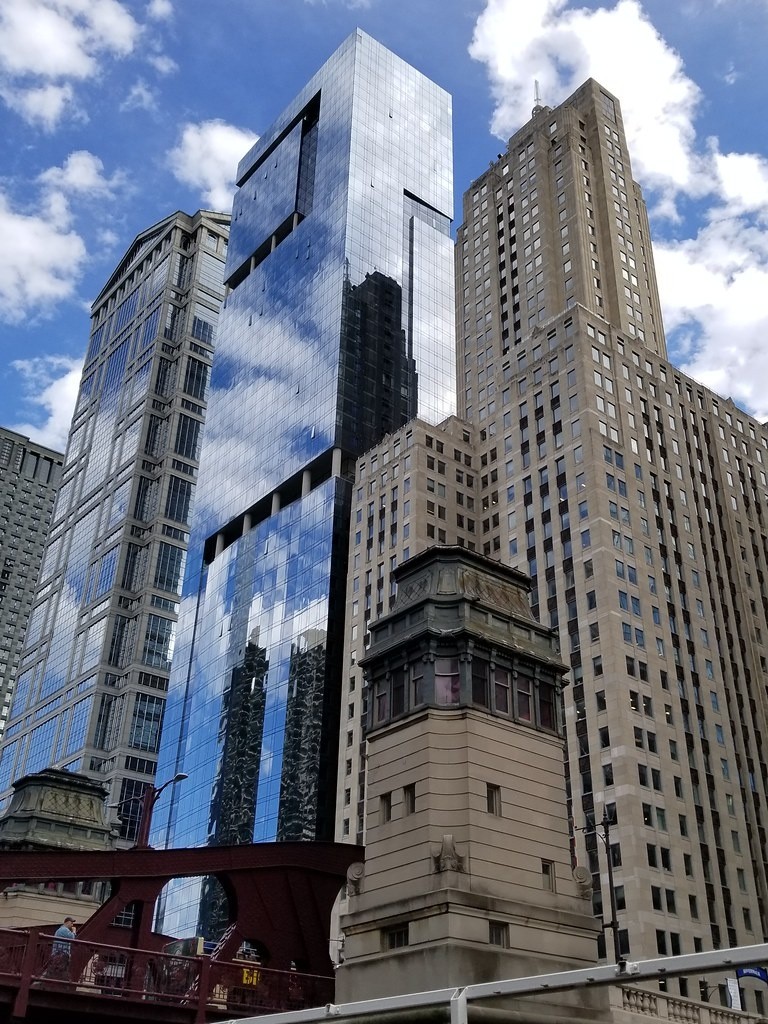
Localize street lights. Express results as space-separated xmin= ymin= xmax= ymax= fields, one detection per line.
xmin=106 ymin=771 xmax=189 ymax=851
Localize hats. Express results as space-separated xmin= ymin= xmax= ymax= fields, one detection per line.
xmin=64 ymin=916 xmax=77 ymax=923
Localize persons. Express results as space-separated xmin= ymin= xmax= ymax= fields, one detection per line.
xmin=32 ymin=917 xmax=77 ymax=985
